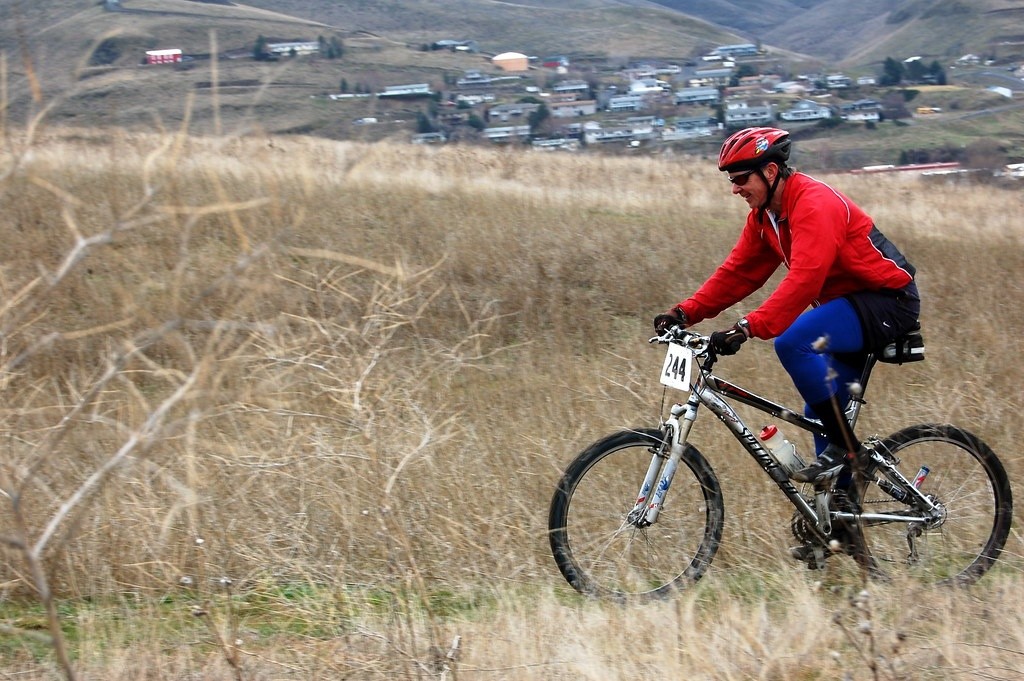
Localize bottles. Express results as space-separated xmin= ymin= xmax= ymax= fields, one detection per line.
xmin=760 ymin=425 xmax=807 ymax=477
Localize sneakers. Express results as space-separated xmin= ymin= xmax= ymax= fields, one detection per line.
xmin=791 ymin=443 xmax=868 ymax=483
xmin=790 ymin=543 xmax=836 ymax=562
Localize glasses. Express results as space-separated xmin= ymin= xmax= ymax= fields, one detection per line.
xmin=727 ymin=168 xmax=762 ymax=186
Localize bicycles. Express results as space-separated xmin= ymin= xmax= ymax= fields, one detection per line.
xmin=545 ymin=318 xmax=1013 ymax=606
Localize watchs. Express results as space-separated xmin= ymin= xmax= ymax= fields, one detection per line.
xmin=739 ymin=319 xmax=751 ymax=336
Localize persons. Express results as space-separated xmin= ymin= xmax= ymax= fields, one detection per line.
xmin=653 ymin=126 xmax=923 ymax=566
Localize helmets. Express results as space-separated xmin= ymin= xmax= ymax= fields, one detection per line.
xmin=719 ymin=127 xmax=789 ymax=172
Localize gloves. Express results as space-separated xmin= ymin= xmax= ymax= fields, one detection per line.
xmin=654 ymin=308 xmax=685 ymax=336
xmin=712 ymin=326 xmax=748 ymax=356
